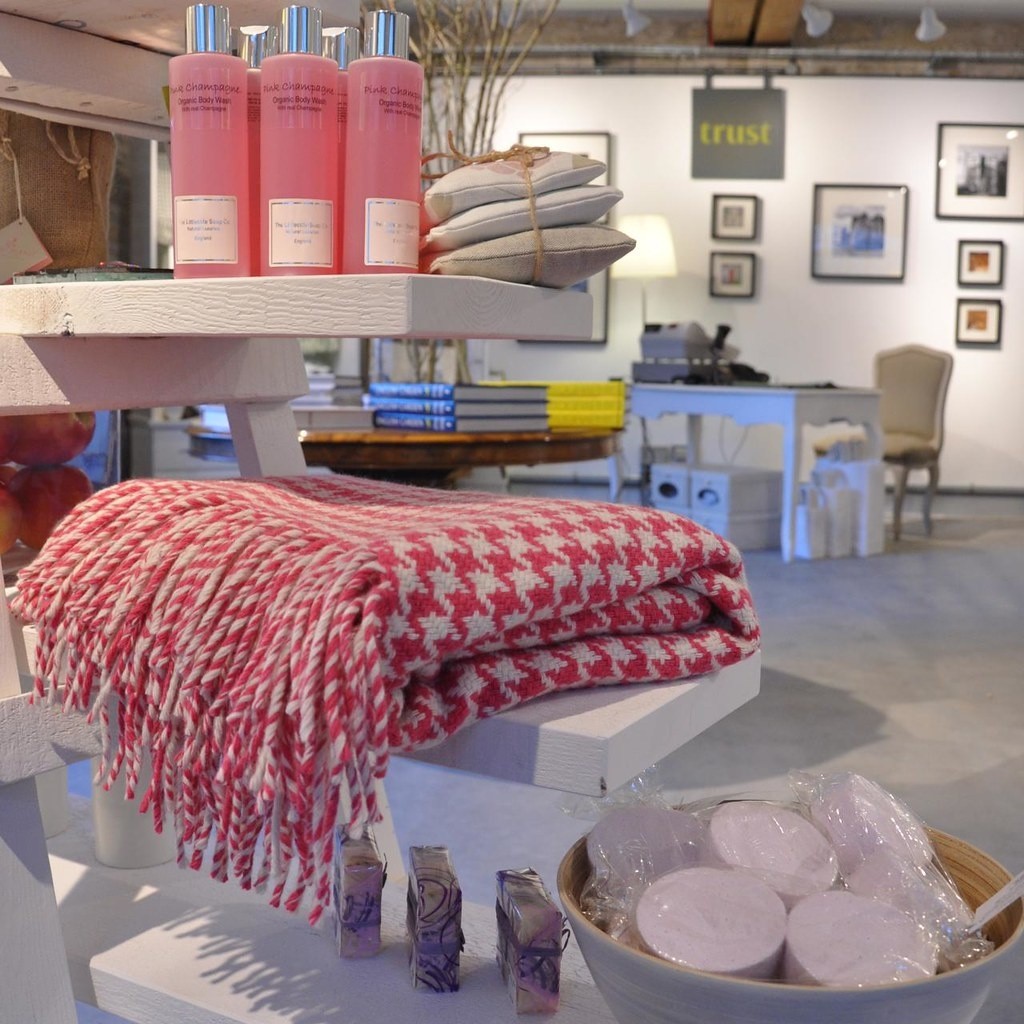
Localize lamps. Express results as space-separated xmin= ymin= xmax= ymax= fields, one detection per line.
xmin=609 ymin=214 xmax=676 ymax=445
xmin=915 ymin=5 xmax=945 ymax=42
xmin=620 ymin=0 xmax=650 ymax=37
xmin=801 ymin=4 xmax=832 ymax=35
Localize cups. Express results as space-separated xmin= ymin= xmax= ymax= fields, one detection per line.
xmin=637 ymin=868 xmax=786 ymax=982
xmin=700 ymin=802 xmax=839 ymax=914
xmin=785 ymin=890 xmax=936 ymax=987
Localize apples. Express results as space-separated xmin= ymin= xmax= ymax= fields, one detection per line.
xmin=0 ymin=412 xmax=96 ymax=557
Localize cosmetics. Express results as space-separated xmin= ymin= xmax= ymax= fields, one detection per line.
xmin=167 ymin=3 xmax=423 ymax=280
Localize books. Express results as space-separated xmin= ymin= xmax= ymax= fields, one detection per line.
xmin=192 ymin=374 xmax=625 ymax=435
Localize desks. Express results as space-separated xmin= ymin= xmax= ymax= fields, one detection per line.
xmin=607 ymin=384 xmax=884 ymax=561
xmin=185 ymin=421 xmax=615 ymax=491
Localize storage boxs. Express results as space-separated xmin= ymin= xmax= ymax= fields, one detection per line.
xmin=649 ymin=460 xmax=711 ymax=506
xmin=655 ymin=504 xmax=692 ymax=518
xmin=691 ymin=467 xmax=782 ymax=515
xmin=691 ymin=515 xmax=780 ymax=550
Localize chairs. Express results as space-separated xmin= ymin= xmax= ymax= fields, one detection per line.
xmin=812 ymin=344 xmax=953 ymax=542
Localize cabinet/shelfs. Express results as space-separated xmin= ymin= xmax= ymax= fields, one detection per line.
xmin=0 ymin=0 xmax=760 ymax=1023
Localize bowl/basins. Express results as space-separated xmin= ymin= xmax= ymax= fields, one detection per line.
xmin=556 ymin=803 xmax=1024 ymax=1024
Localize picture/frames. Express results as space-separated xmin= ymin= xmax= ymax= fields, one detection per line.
xmin=958 ymin=239 xmax=1004 ymax=286
xmin=955 ymin=297 xmax=1001 ymax=345
xmin=935 ymin=120 xmax=1024 ymax=223
xmin=810 ymin=183 xmax=907 ymax=280
xmin=709 ymin=252 xmax=756 ymax=299
xmin=517 ymin=130 xmax=611 ymax=344
xmin=710 ymin=193 xmax=757 ymax=239
